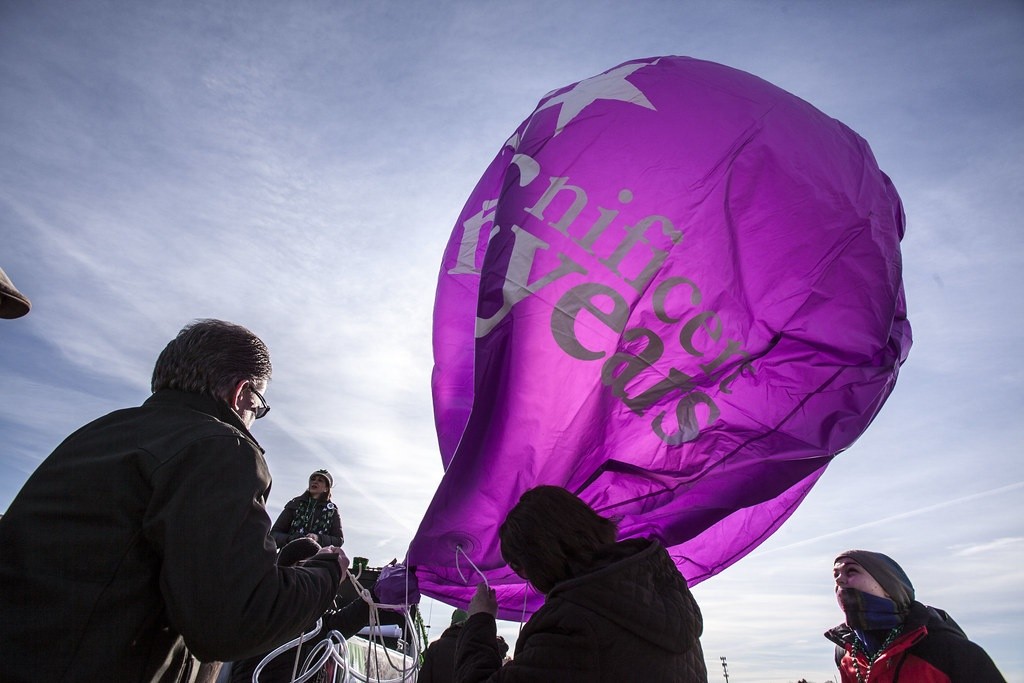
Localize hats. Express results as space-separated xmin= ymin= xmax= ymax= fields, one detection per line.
xmin=0 ymin=264 xmax=31 ymax=319
xmin=310 ymin=469 xmax=333 ymax=489
xmin=450 ymin=608 xmax=469 ymax=625
xmin=834 ymin=550 xmax=915 ymax=607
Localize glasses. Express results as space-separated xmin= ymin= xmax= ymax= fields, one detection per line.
xmin=236 ymin=379 xmax=270 ymax=419
xmin=509 ymin=561 xmax=530 ymax=580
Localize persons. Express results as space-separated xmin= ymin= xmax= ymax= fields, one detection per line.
xmin=236 ymin=538 xmax=398 ymax=682
xmin=417 ymin=607 xmax=473 ymax=682
xmin=2 ymin=317 xmax=351 ymax=683
xmin=444 ymin=485 xmax=709 ymax=683
xmin=822 ymin=550 xmax=1009 ymax=683
xmin=270 ymin=467 xmax=349 ymax=568
